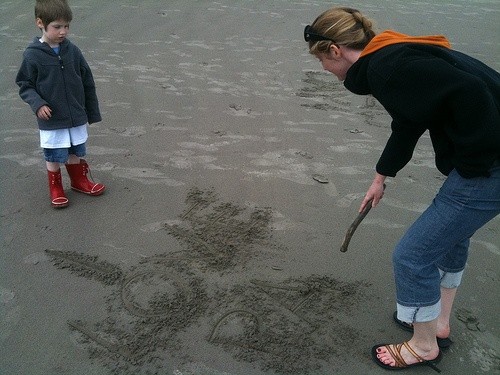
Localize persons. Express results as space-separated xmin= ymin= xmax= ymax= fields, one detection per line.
xmin=15 ymin=0 xmax=105 ymax=210
xmin=304 ymin=7 xmax=500 ymax=373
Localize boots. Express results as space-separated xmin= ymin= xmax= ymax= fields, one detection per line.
xmin=64 ymin=159 xmax=105 ymax=195
xmin=46 ymin=167 xmax=69 ymax=208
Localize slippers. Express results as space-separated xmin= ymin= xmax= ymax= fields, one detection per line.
xmin=371 ymin=341 xmax=442 ymax=370
xmin=393 ymin=311 xmax=451 ymax=348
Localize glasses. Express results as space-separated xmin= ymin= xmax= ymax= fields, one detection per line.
xmin=304 ymin=25 xmax=340 ymax=49
